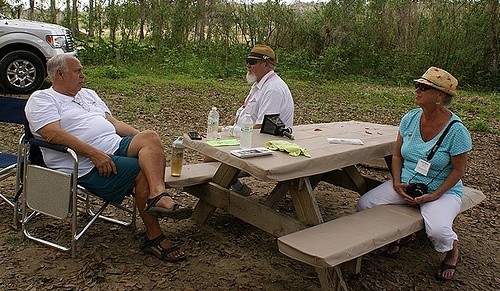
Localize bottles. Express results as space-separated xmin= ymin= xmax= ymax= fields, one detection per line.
xmin=171 ymin=136 xmax=185 ymax=177
xmin=221 ymin=126 xmax=241 ymax=137
xmin=206 ymin=107 xmax=219 ymax=141
xmin=240 ymin=113 xmax=253 ymax=150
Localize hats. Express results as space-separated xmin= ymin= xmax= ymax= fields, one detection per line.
xmin=414 ymin=66 xmax=458 ymax=97
xmin=243 ymin=44 xmax=276 ymax=66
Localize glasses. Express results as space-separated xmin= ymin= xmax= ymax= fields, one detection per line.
xmin=415 ymin=82 xmax=439 ymax=91
xmin=244 ymin=59 xmax=263 ymax=66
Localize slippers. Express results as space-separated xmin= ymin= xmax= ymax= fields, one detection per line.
xmin=435 ymin=251 xmax=461 ymax=280
xmin=383 ymin=233 xmax=412 ymax=257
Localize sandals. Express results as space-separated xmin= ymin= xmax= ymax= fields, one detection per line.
xmin=143 ymin=191 xmax=193 ymax=219
xmin=138 ymin=233 xmax=187 ymax=263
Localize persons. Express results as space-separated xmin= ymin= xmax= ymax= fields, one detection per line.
xmin=203 ymin=44 xmax=294 ymax=195
xmin=25 ymin=54 xmax=193 ymax=262
xmin=357 ymin=67 xmax=472 ymax=280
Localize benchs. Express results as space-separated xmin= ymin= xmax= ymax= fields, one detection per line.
xmin=277 ymin=184 xmax=486 ymax=291
xmin=161 ymin=162 xmax=255 ymax=227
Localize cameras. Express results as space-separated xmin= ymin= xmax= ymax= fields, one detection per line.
xmin=406 ymin=183 xmax=428 ymax=198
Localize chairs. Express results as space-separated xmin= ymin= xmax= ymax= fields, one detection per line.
xmin=0 ymin=97 xmax=137 ymax=258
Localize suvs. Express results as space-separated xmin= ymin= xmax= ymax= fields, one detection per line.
xmin=0 ymin=14 xmax=78 ymax=94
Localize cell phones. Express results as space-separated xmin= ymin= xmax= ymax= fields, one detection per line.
xmin=189 ymin=132 xmax=202 ymax=140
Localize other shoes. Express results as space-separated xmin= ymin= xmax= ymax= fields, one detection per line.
xmin=228 ymin=179 xmax=251 ymax=195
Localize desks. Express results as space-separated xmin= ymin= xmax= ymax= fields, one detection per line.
xmin=183 ymin=120 xmax=400 ymax=291
